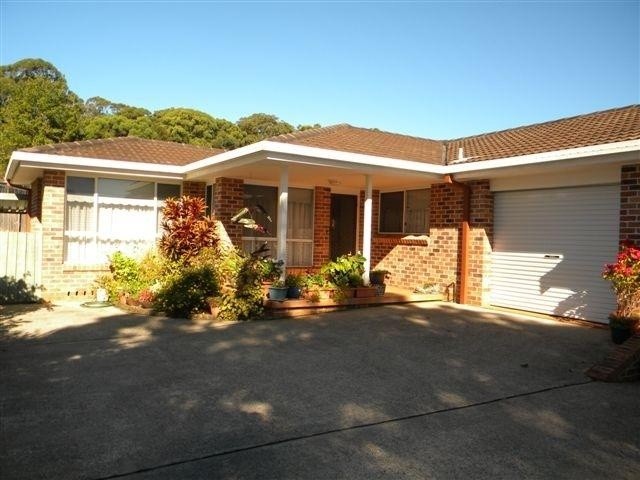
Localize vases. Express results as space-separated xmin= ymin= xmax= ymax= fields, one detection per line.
xmin=611 ymin=327 xmax=633 ymax=345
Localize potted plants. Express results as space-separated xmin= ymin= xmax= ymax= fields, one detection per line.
xmin=204 ymin=294 xmax=221 ymax=315
xmin=267 ymin=250 xmax=392 ymax=305
xmin=88 ymin=244 xmax=164 ymax=308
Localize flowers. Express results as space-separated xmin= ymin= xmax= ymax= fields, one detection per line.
xmin=600 ymin=238 xmax=640 ymax=334
